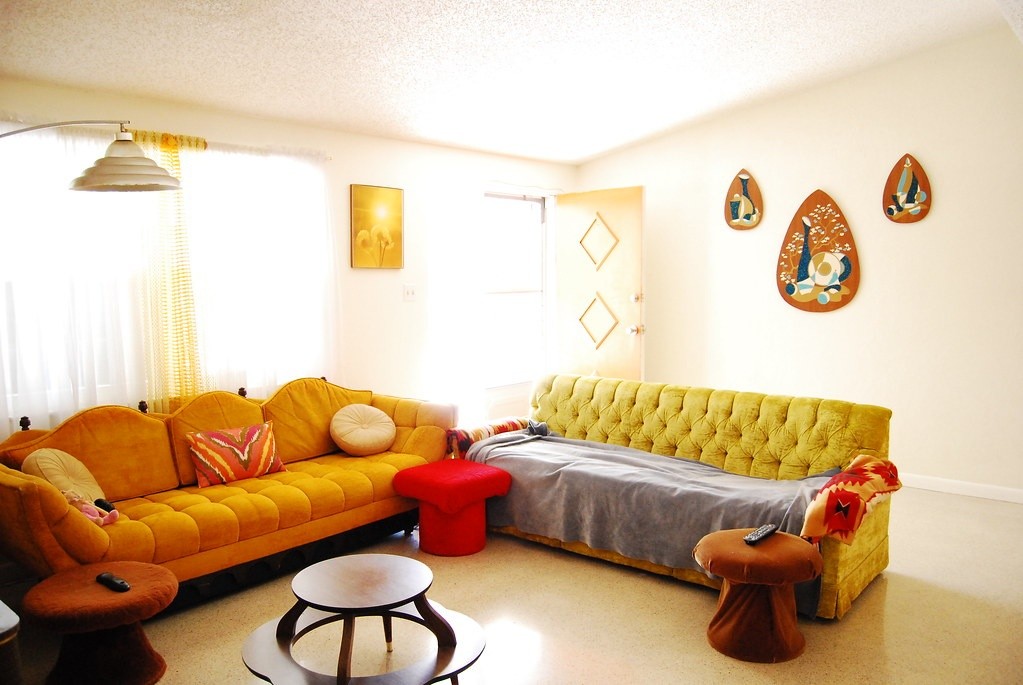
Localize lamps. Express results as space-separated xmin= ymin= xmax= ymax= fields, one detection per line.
xmin=0 ymin=119 xmax=183 ymax=191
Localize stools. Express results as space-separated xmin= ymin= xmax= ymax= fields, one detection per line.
xmin=391 ymin=460 xmax=511 ymax=557
xmin=690 ymin=528 xmax=826 ymax=662
xmin=22 ymin=561 xmax=179 ymax=685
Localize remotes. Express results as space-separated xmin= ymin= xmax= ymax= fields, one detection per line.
xmin=743 ymin=524 xmax=777 ymax=545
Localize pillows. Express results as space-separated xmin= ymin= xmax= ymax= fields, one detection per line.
xmin=330 ymin=403 xmax=398 ymax=457
xmin=25 ymin=446 xmax=106 ymax=514
xmin=183 ymin=419 xmax=285 ymax=487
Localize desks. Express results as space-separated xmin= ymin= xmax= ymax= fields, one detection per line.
xmin=243 ymin=552 xmax=487 ymax=685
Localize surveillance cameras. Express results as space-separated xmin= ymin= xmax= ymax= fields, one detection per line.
xmin=96 ymin=571 xmax=131 ymax=592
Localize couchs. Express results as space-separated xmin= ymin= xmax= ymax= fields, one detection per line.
xmin=444 ymin=372 xmax=891 ymax=617
xmin=0 ymin=378 xmax=456 ymax=582
